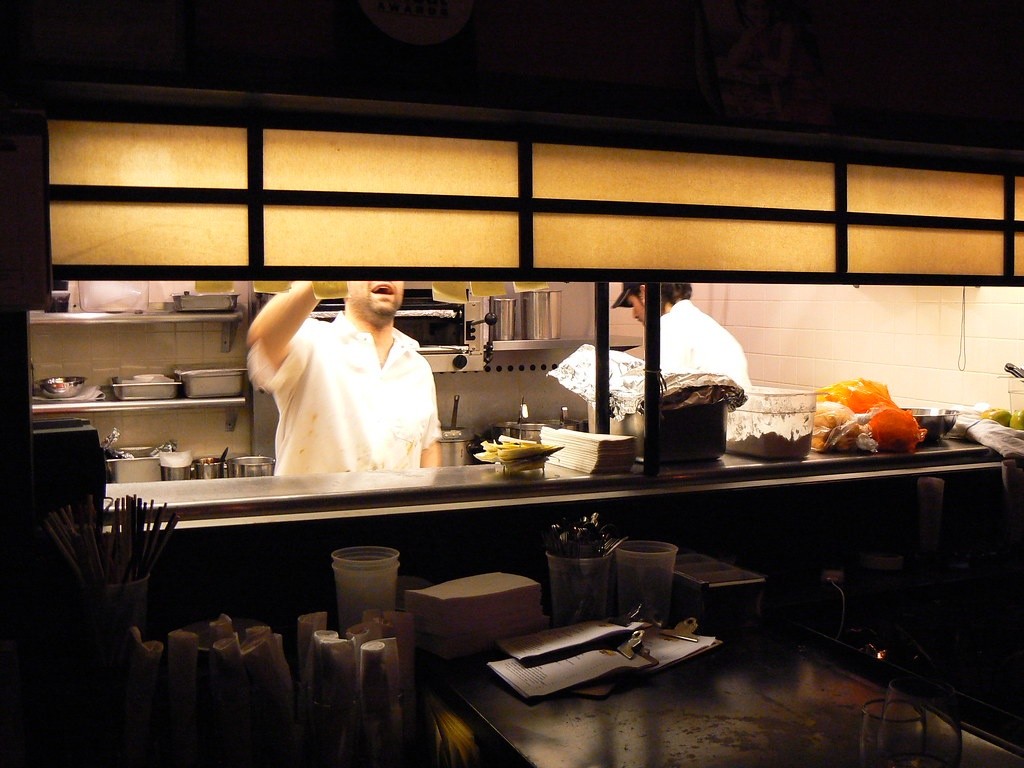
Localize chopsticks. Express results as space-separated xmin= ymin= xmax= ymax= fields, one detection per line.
xmin=43 ymin=494 xmax=181 ymax=586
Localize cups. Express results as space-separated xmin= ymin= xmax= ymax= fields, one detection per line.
xmin=81 ymin=573 xmax=149 ymax=663
xmin=859 ymin=678 xmax=963 ymax=768
xmin=546 ymin=548 xmax=613 ymax=628
xmin=331 ymin=546 xmax=401 ymax=638
xmin=616 ymin=540 xmax=679 ymax=627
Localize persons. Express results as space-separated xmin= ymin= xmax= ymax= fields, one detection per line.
xmin=610 ymin=283 xmax=754 ymax=393
xmin=244 ymin=280 xmax=444 ymax=477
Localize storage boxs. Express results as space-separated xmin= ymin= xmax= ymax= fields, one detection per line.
xmin=725 ymin=384 xmax=830 ymax=463
xmin=671 ymin=552 xmax=767 ymax=639
xmin=587 ymin=395 xmax=727 ymax=468
xmin=174 ymin=368 xmax=245 ymax=399
xmin=112 ymin=381 xmax=182 ymax=400
xmin=172 ymin=294 xmax=240 ymax=311
xmin=106 ymin=458 xmax=162 ymax=482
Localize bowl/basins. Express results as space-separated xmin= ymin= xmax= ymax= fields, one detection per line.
xmin=903 ymin=407 xmax=962 ymax=443
xmin=33 ymin=376 xmax=88 ymax=399
xmin=119 ymin=447 xmax=154 ymax=458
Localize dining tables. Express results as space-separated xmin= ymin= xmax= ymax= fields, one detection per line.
xmin=404 ymin=612 xmax=1024 ymax=767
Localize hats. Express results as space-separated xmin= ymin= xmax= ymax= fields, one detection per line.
xmin=612 ymin=280 xmax=644 ymax=311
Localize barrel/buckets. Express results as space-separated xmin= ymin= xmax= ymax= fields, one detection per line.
xmin=161 ymin=462 xmax=193 ymax=481
xmin=192 ymin=458 xmax=227 ymax=479
xmin=488 ymin=418 xmax=589 ymax=441
xmin=440 ymin=426 xmax=480 ymax=467
xmin=493 ymin=298 xmax=516 ymax=341
xmin=227 ymin=456 xmax=275 ymax=478
xmin=519 ymin=291 xmax=561 ymax=340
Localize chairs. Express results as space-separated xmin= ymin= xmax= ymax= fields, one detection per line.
xmin=817 ymin=475 xmax=945 ymax=676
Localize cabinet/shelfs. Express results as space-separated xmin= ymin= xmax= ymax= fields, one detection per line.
xmin=28 ymin=281 xmax=257 ymax=482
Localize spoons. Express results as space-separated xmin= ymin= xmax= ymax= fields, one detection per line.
xmin=543 ymin=511 xmax=628 ymax=560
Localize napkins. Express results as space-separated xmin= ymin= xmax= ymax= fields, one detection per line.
xmin=539 ymin=426 xmax=637 ymax=475
